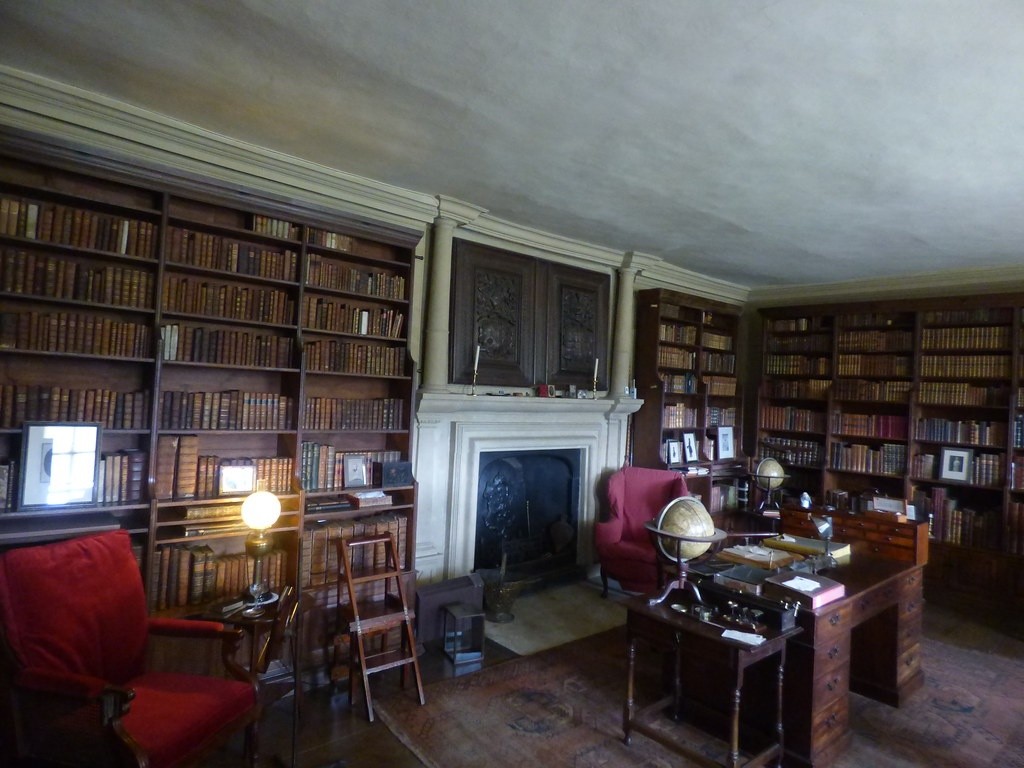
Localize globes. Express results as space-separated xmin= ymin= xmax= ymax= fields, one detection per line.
xmin=643 ymin=496 xmax=728 ymax=607
xmin=747 ymin=458 xmax=791 ymax=511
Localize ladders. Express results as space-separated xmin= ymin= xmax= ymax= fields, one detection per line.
xmin=335 ymin=530 xmax=425 ymax=723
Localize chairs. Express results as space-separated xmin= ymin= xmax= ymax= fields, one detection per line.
xmin=596 ymin=469 xmax=722 ymax=597
xmin=0 ymin=528 xmax=265 ymax=767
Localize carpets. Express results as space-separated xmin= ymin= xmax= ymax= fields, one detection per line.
xmin=369 ymin=622 xmax=1024 ymax=767
xmin=485 ymin=579 xmax=627 ymax=657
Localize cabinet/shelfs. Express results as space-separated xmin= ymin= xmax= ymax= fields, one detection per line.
xmin=636 ymin=289 xmax=747 ymax=512
xmin=1 ymin=146 xmax=414 ymax=695
xmin=780 ymin=503 xmax=930 ymax=567
xmin=752 ymin=290 xmax=1024 ymax=581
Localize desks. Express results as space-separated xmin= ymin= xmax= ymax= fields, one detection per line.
xmin=623 ymin=605 xmax=804 ymax=768
xmin=214 ymin=591 xmax=313 ymax=768
xmin=700 ymin=552 xmax=930 ymax=768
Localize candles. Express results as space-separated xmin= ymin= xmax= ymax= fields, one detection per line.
xmin=472 ymin=345 xmax=481 ymax=372
xmin=593 ymin=358 xmax=599 ymax=381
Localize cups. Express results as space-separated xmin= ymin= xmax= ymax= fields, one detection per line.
xmin=700 ymin=607 xmax=712 ymax=623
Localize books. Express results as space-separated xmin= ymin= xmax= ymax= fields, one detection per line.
xmin=0 ymin=195 xmax=405 ymax=612
xmin=655 ymin=307 xmax=1024 ymax=544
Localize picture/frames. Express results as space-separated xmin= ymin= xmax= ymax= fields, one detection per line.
xmin=716 ymin=424 xmax=735 ymax=461
xmin=15 ymin=421 xmax=102 ymax=512
xmin=681 ymin=431 xmax=699 ymax=463
xmin=667 ymin=440 xmax=681 ymax=466
xmin=342 ymin=454 xmax=369 ymax=488
xmin=218 ymin=465 xmax=257 ymax=497
xmin=937 ymin=446 xmax=975 ymax=486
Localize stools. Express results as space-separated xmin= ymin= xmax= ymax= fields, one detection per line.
xmin=441 ymin=602 xmax=489 ymax=665
